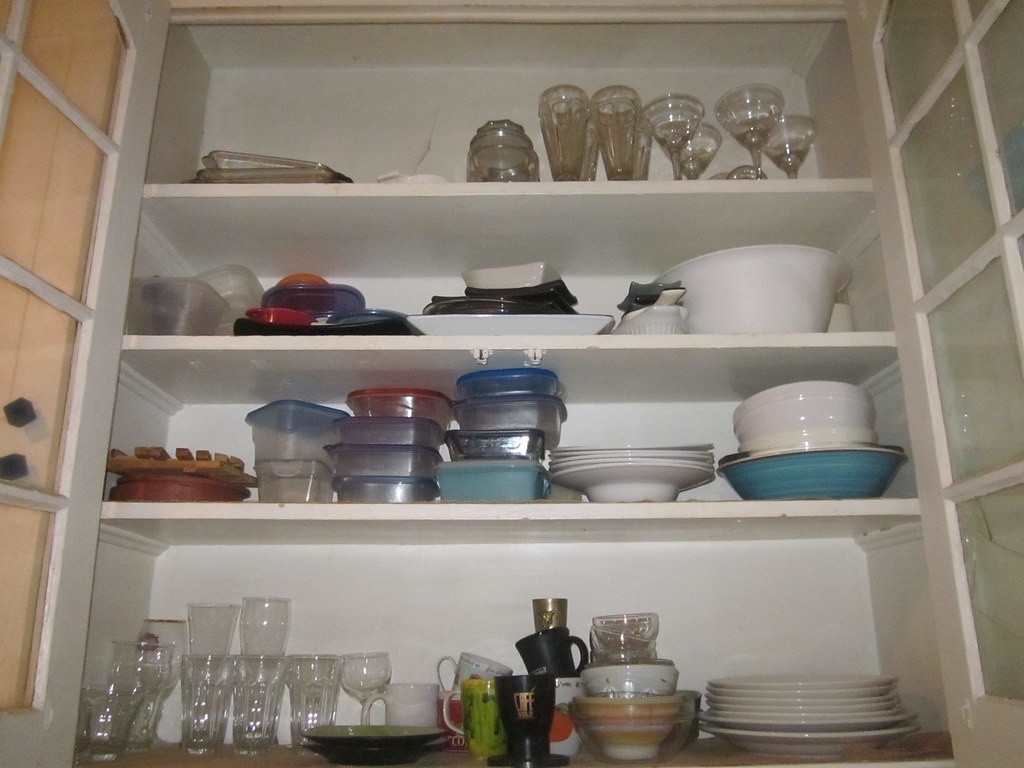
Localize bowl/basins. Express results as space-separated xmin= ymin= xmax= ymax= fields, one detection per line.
xmin=717 ymin=441 xmax=909 ymax=500
xmin=122 ymin=264 xmax=414 ymax=335
xmin=567 ymin=611 xmax=702 ymax=767
xmin=547 ymin=441 xmax=717 ymax=504
xmin=732 ymin=380 xmax=877 ymax=454
xmin=651 ymin=243 xmax=853 ymax=335
xmin=461 ymin=261 xmax=561 ymax=289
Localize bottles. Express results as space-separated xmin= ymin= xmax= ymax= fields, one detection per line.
xmin=466 ymin=119 xmax=541 ymax=182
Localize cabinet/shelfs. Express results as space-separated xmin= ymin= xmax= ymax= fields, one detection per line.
xmin=75 ymin=9 xmax=953 ymax=766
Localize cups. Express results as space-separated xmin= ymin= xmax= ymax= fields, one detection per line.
xmin=359 ymin=597 xmax=589 ymax=768
xmin=73 ymin=595 xmax=346 ymax=768
xmin=538 ymin=84 xmax=654 ymax=182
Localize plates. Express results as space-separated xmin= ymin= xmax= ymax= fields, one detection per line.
xmin=404 ymin=279 xmax=616 ymax=336
xmin=189 ymin=148 xmax=354 ymax=184
xmin=606 ymin=280 xmax=689 ymax=335
xmin=298 ymin=724 xmax=454 ymax=766
xmin=697 ymin=670 xmax=921 ymax=760
xmin=232 ymin=316 xmax=412 ymax=336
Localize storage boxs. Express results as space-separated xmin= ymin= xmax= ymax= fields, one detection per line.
xmin=126 ymin=268 xmax=227 ymax=337
xmin=322 ymin=388 xmax=453 ymax=503
xmin=190 ymin=264 xmax=267 ymax=316
xmin=436 ymin=368 xmax=569 ymax=503
xmin=262 ymin=282 xmax=365 ymax=317
xmin=244 ymin=392 xmax=349 ymax=502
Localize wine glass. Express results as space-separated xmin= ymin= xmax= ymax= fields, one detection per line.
xmin=641 ymin=83 xmax=820 ymax=181
xmin=135 ymin=617 xmax=188 ymax=746
xmin=338 ymin=651 xmax=392 ymax=723
